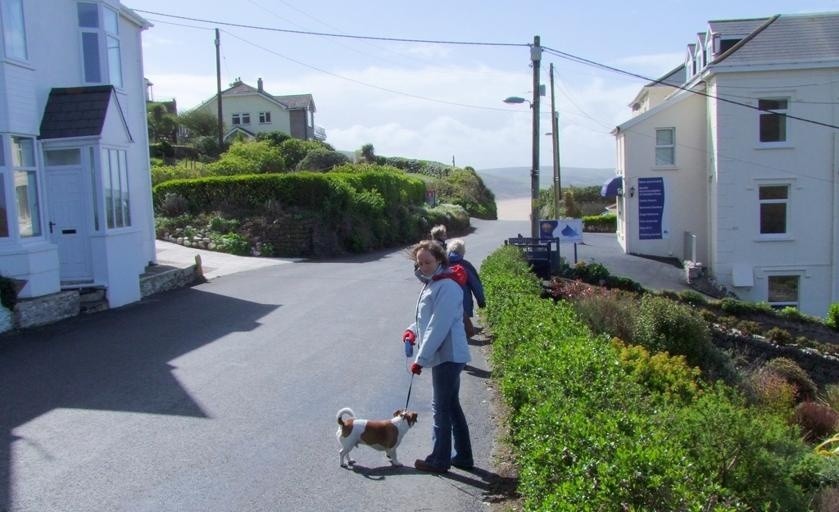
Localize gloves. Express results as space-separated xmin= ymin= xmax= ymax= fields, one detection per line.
xmin=411 ymin=363 xmax=423 ymax=375
xmin=403 ymin=330 xmax=416 ymax=345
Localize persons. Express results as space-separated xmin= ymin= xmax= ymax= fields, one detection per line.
xmin=401 ymin=241 xmax=475 ymax=473
xmin=427 ymin=223 xmax=448 ymax=254
xmin=446 ymin=239 xmax=487 ymax=337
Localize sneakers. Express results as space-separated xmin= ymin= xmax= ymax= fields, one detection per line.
xmin=450 ymin=456 xmax=472 ymax=469
xmin=415 ymin=459 xmax=447 ymax=473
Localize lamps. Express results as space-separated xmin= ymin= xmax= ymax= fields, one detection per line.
xmin=629 ymin=186 xmax=635 ymax=197
xmin=617 ymin=186 xmax=622 ymax=197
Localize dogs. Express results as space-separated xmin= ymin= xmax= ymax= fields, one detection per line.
xmin=335 ymin=407 xmax=418 ymax=468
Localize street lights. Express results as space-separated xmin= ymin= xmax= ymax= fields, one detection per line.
xmin=501 ymin=34 xmax=543 ymax=253
